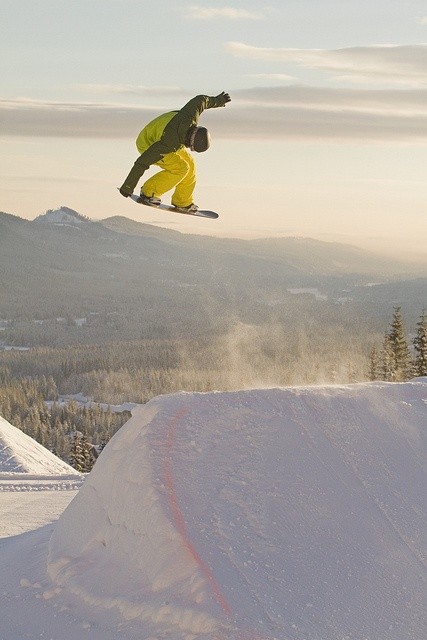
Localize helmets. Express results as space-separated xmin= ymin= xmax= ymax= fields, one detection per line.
xmin=190 ymin=126 xmax=211 ymax=152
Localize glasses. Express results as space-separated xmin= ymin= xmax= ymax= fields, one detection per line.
xmin=188 ymin=144 xmax=193 ymax=152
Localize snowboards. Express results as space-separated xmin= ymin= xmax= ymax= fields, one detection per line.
xmin=116 ymin=187 xmax=218 ymax=219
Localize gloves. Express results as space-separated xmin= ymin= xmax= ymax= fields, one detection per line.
xmin=119 ymin=183 xmax=133 ymax=198
xmin=208 ymin=91 xmax=230 ymax=110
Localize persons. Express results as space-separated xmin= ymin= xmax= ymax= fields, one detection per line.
xmin=120 ymin=91 xmax=232 ymax=214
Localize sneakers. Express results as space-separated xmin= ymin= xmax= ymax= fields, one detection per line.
xmin=139 ymin=193 xmax=161 ymax=206
xmin=174 ymin=203 xmax=200 ymax=214
